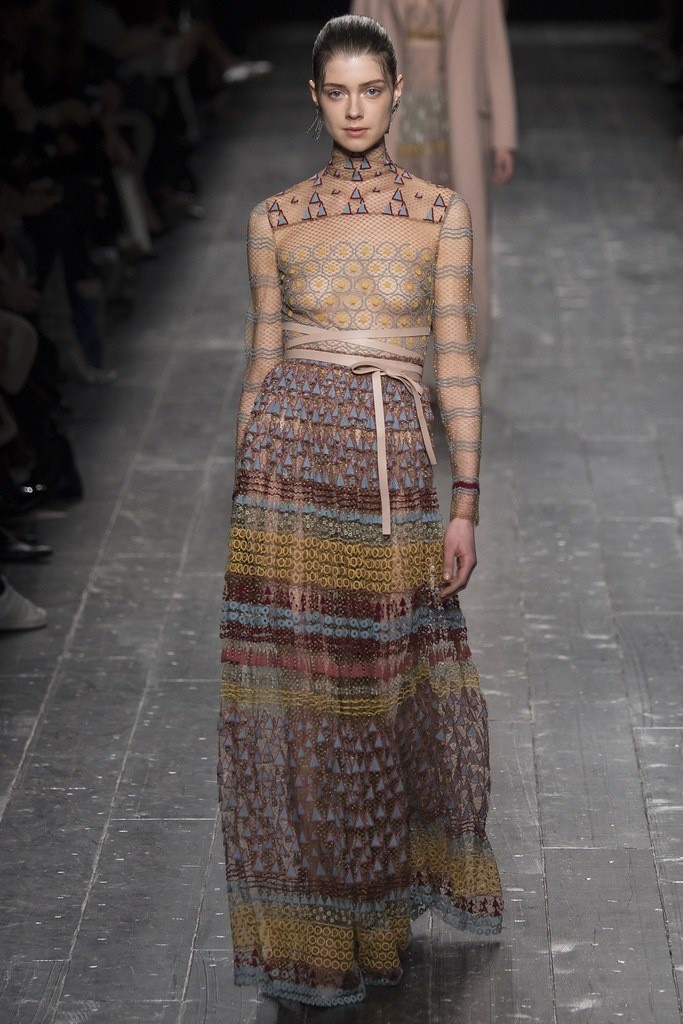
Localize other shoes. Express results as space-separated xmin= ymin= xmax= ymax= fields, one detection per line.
xmin=72 ymin=366 xmax=117 ymax=384
xmin=0 ymin=527 xmax=52 ymax=558
xmin=0 ymin=479 xmax=50 ymax=514
xmin=0 ymin=575 xmax=47 ymax=630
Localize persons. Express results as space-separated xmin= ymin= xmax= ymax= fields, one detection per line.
xmin=215 ymin=16 xmax=504 ymax=1004
xmin=349 ymin=0 xmax=518 ymax=403
xmin=0 ymin=0 xmax=243 ymax=629
